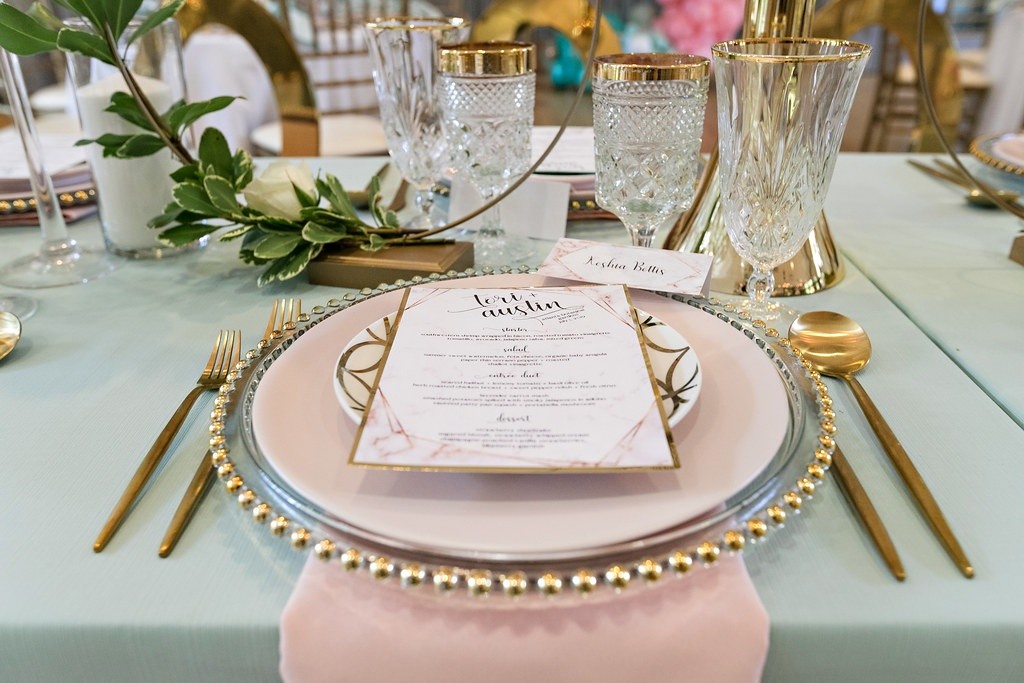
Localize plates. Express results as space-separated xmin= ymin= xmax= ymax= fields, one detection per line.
xmin=0 ymin=111 xmax=97 ymax=213
xmin=969 ymin=128 xmax=1024 ymax=176
xmin=434 ymin=125 xmax=599 ymax=212
xmin=208 ymin=263 xmax=837 ymax=600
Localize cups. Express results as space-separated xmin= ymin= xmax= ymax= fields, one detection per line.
xmin=62 ymin=14 xmax=210 ymax=260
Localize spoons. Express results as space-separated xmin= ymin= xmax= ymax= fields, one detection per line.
xmin=787 ymin=310 xmax=974 ymax=578
xmin=905 ymin=157 xmax=1019 ymax=207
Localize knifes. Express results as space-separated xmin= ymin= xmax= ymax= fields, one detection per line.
xmin=771 ymin=341 xmax=906 ymax=582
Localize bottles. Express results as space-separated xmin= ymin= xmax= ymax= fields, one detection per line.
xmin=660 ymin=0 xmax=846 ymax=299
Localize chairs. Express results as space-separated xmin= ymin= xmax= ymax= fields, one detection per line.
xmin=123 ymin=1 xmax=964 ymax=155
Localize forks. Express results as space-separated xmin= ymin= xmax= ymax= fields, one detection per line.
xmin=159 ymin=297 xmax=302 ymax=557
xmin=94 ymin=329 xmax=242 ymax=552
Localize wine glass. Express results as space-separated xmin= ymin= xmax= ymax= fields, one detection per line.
xmin=432 ymin=41 xmax=537 ymax=267
xmin=710 ymin=37 xmax=874 ymax=337
xmin=0 ymin=46 xmax=128 ymax=290
xmin=362 ymin=13 xmax=472 ymax=242
xmin=593 ymin=52 xmax=712 ymax=249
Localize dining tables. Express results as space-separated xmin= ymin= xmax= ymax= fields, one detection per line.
xmin=0 ymin=141 xmax=1023 ymax=682
